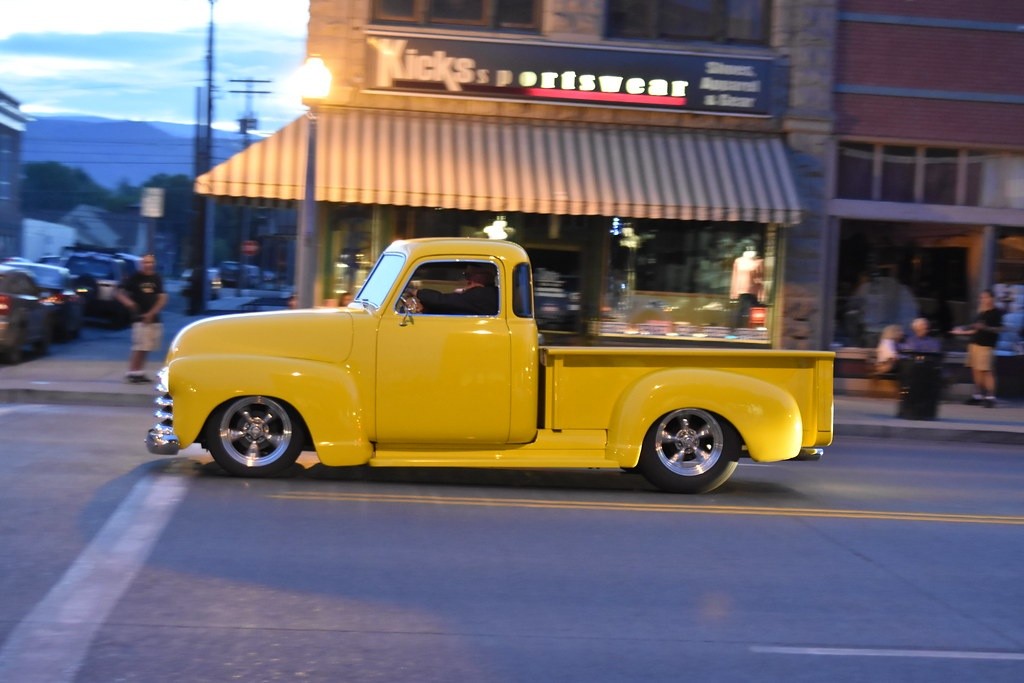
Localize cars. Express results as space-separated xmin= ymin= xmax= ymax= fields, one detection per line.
xmin=179 ymin=260 xmax=276 ymax=298
xmin=0 ymin=248 xmax=144 ymax=365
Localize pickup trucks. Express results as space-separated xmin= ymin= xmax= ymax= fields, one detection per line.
xmin=144 ymin=236 xmax=836 ymax=494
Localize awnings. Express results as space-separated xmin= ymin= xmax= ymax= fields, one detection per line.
xmin=194 ymin=112 xmax=803 ymax=227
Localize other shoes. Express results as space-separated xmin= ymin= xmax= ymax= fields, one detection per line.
xmin=968 ymin=394 xmax=982 ymax=404
xmin=128 ymin=371 xmax=148 ymax=382
xmin=985 ymin=396 xmax=994 ymax=407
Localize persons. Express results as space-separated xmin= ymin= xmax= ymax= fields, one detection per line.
xmin=950 ymin=290 xmax=1008 ymax=408
xmin=404 ymin=263 xmax=499 ymax=316
xmin=113 ymin=255 xmax=168 ymax=385
xmin=876 ymin=317 xmax=953 ymax=402
xmin=729 ymin=250 xmax=765 ymax=330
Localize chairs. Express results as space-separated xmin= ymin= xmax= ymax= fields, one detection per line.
xmin=868 ymin=352 xmax=906 ymax=411
